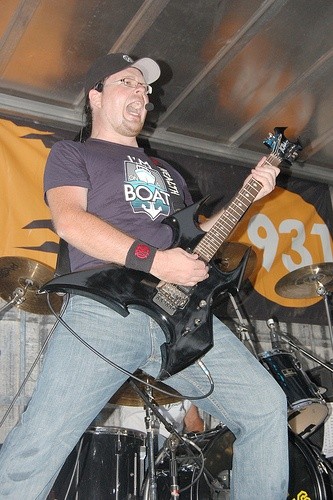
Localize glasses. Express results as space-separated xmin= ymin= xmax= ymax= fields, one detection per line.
xmin=101 ymin=77 xmax=153 ymax=95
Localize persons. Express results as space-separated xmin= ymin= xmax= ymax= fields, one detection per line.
xmin=100 ymin=400 xmax=207 ymax=451
xmin=0 ymin=54 xmax=287 ymax=500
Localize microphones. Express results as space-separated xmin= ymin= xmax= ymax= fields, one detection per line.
xmin=145 ymin=103 xmax=155 ymax=110
xmin=267 ymin=317 xmax=281 ymax=349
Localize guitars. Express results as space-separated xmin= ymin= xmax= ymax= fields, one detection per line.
xmin=38 ymin=126 xmax=305 ymax=383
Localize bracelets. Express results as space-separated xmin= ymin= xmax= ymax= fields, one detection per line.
xmin=125 ymin=239 xmax=158 ymax=273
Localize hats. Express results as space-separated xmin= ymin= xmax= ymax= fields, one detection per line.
xmin=84 ymin=53 xmax=161 ymax=101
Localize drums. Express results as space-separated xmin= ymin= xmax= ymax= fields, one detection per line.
xmin=54 ymin=426 xmax=147 ymax=500
xmin=253 ymin=350 xmax=330 ymax=439
xmin=152 ymin=426 xmax=333 ymax=500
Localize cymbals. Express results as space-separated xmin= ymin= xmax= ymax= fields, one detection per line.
xmin=0 ymin=256 xmax=64 ymax=314
xmin=275 ymin=262 xmax=333 ymax=298
xmin=214 ymin=240 xmax=256 ymax=278
xmin=105 ymin=364 xmax=187 ymax=406
